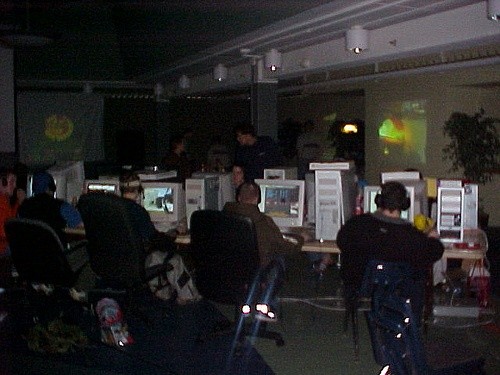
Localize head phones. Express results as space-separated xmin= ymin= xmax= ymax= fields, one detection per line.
xmin=235 ymin=180 xmax=261 ymax=204
xmin=118 ymin=172 xmax=142 ymax=192
xmin=32 ymin=171 xmax=56 ymax=193
xmin=375 ymin=181 xmax=411 ymax=210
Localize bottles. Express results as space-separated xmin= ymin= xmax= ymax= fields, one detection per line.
xmin=72 ymin=197 xmax=77 ymax=207
xmin=352 ymin=198 xmax=363 ymax=216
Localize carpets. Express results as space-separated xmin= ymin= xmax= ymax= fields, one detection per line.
xmin=0 ymin=271 xmax=276 ymax=375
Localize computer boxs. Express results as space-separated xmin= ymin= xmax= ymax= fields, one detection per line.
xmin=315 ymin=168 xmax=354 ymax=242
xmin=264 ymin=165 xmax=298 ymax=181
xmin=437 ymin=184 xmax=479 ymax=243
xmin=185 ymin=175 xmax=219 ymax=231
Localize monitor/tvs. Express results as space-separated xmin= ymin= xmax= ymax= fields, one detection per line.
xmin=138 ymin=182 xmax=186 ymax=222
xmin=362 ymin=184 xmax=415 ymax=228
xmin=85 ymin=178 xmax=119 ymax=195
xmin=254 ymin=179 xmax=307 ymax=229
xmin=25 ymin=173 xmax=57 ymax=199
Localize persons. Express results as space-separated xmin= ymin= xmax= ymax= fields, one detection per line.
xmin=336 ymin=179 xmax=445 ymax=332
xmin=1 ymin=167 xmax=203 ymax=307
xmin=296 ymin=120 xmax=325 ymax=179
xmin=169 ymin=122 xmax=282 ymax=196
xmin=222 ymin=179 xmax=298 ymax=266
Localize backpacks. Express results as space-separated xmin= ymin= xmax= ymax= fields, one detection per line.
xmin=144 ymin=250 xmax=203 ymax=304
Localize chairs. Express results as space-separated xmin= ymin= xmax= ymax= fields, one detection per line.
xmin=345 ymin=260 xmax=488 ymax=374
xmin=77 ymin=191 xmax=175 ymax=327
xmin=190 ymin=209 xmax=285 ymax=349
xmin=3 ymin=218 xmax=87 ymax=321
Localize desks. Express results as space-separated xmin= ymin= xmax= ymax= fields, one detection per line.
xmin=65 ymin=225 xmax=482 ymax=260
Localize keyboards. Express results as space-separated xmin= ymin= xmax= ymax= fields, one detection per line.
xmin=154 ymin=223 xmax=176 ymax=233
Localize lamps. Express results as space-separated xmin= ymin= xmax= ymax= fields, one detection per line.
xmin=214 ymin=64 xmax=226 ymax=81
xmin=346 ymin=25 xmax=369 ymax=53
xmin=178 ymin=75 xmax=189 ymax=89
xmin=487 ymin=0 xmax=500 ymax=20
xmin=264 ymin=48 xmax=282 ymax=70
